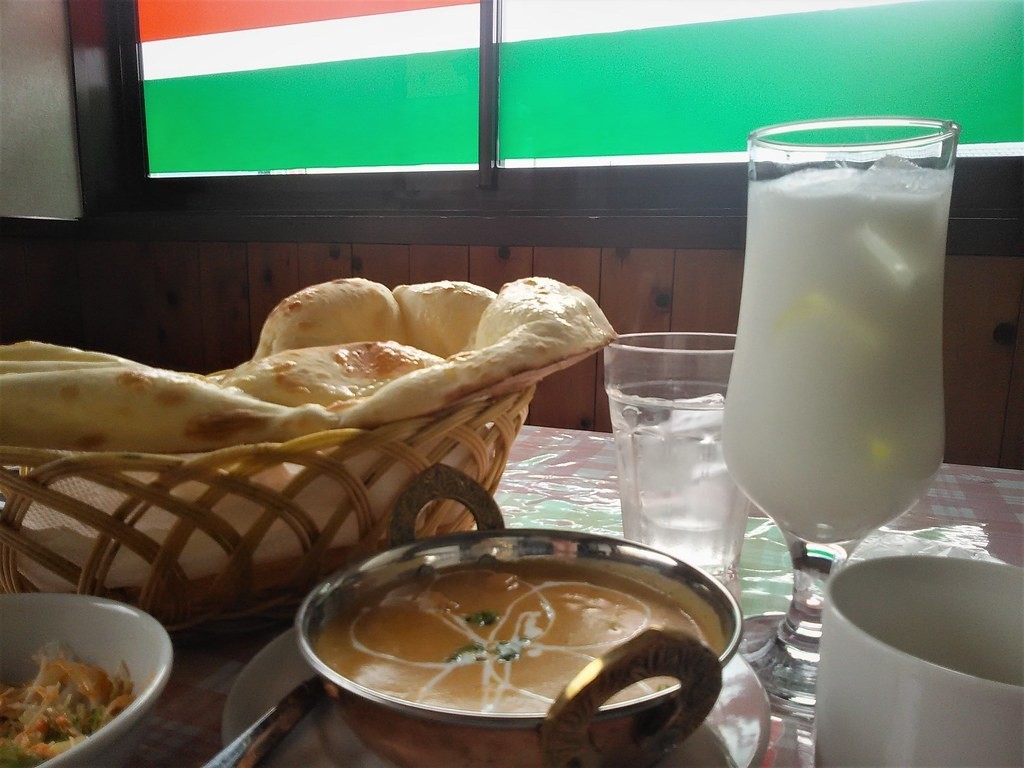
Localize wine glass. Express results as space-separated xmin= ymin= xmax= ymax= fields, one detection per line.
xmin=721 ymin=117 xmax=962 ymax=718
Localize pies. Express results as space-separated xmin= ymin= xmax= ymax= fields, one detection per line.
xmin=0 ymin=275 xmax=614 ymax=456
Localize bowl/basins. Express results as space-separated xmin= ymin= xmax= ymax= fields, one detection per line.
xmin=0 ymin=592 xmax=174 ymax=768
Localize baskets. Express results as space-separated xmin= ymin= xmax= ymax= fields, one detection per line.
xmin=0 ymin=385 xmax=543 ymax=638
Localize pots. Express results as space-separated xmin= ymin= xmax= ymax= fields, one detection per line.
xmin=294 ymin=462 xmax=744 ymax=768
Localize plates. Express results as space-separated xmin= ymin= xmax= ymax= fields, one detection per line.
xmin=221 ymin=627 xmax=771 ymax=768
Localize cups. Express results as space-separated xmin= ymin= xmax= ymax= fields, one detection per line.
xmin=604 ymin=332 xmax=750 ymax=586
xmin=814 ymin=555 xmax=1024 ymax=768
